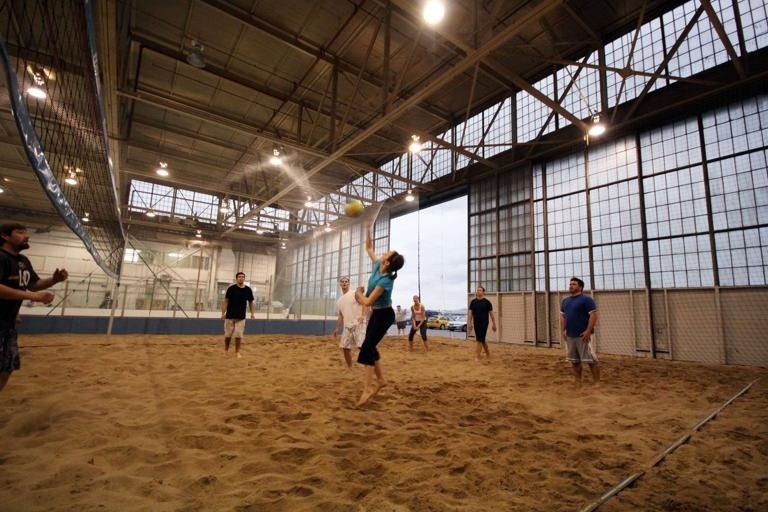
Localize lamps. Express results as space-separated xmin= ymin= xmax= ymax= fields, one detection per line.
xmin=27 ymin=71 xmax=48 ymax=99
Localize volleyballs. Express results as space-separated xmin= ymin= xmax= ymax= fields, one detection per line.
xmin=344 ymin=200 xmax=364 ymax=217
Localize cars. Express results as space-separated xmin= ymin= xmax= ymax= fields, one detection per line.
xmin=406 ymin=311 xmax=467 ymax=333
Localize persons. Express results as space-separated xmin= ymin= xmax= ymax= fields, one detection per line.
xmin=467 ymin=286 xmax=498 ymax=361
xmin=394 ymin=305 xmax=408 ymax=336
xmin=406 ymin=295 xmax=430 ymax=352
xmin=219 ymin=272 xmax=256 ymax=358
xmin=0 ymin=220 xmax=68 ymax=393
xmin=98 ymin=292 xmax=113 ymax=308
xmin=352 ymin=200 xmax=405 ymax=407
xmin=333 ymin=277 xmax=367 ymax=371
xmin=560 ymin=276 xmax=602 ymax=389
